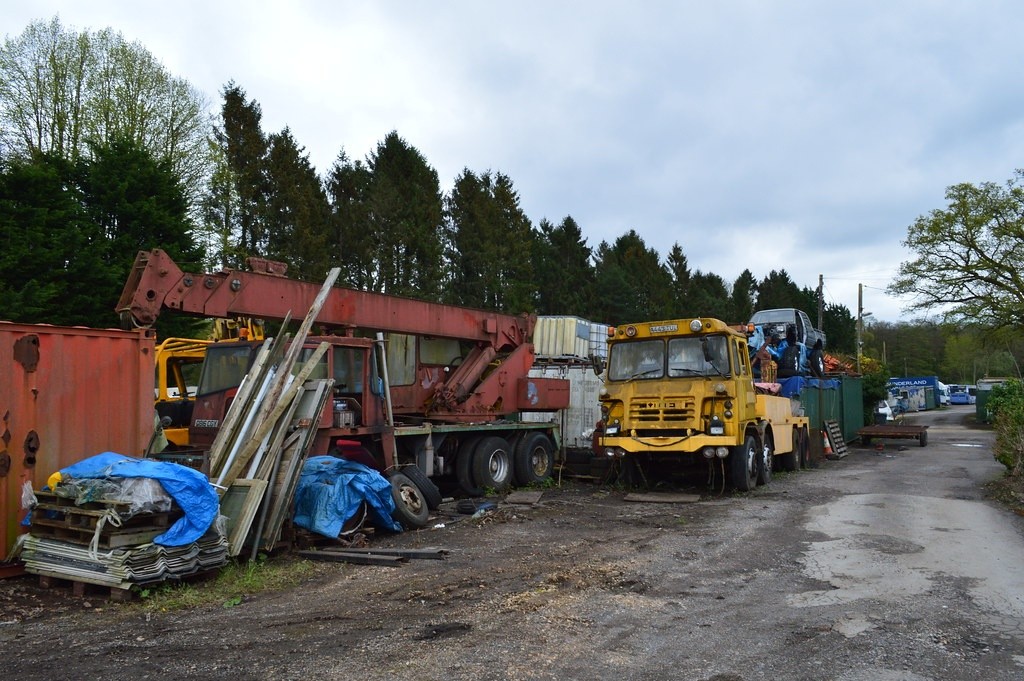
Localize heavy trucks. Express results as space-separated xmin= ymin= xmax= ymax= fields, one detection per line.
xmin=591 ymin=316 xmax=811 ymax=494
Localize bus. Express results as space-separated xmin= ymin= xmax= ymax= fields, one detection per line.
xmin=946 ymin=385 xmax=977 ymax=405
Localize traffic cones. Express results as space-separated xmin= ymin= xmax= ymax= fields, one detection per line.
xmin=824 ymin=430 xmax=838 ymax=461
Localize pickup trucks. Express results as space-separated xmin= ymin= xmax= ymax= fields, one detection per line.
xmin=746 ymin=307 xmax=827 ymax=378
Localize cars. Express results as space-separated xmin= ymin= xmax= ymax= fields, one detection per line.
xmin=879 ymin=400 xmax=894 ymax=421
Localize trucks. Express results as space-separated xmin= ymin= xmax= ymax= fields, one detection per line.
xmin=937 ymin=382 xmax=951 ymax=404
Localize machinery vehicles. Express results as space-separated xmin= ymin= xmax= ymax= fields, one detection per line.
xmin=154 ymin=315 xmax=264 ymax=453
xmin=113 ymin=246 xmax=573 ymax=498
